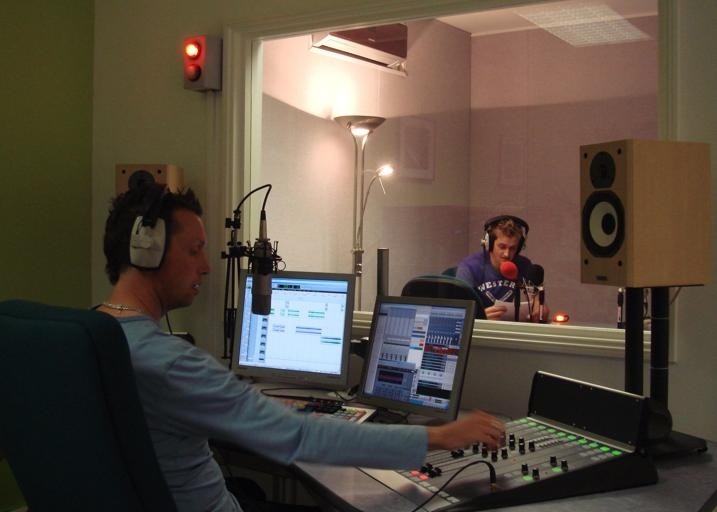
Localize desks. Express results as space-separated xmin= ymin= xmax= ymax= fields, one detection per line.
xmin=209 ymin=376 xmax=717 ymax=512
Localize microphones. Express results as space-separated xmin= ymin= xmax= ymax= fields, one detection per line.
xmin=499 ymin=258 xmax=545 ymax=287
xmin=248 ymin=213 xmax=281 ymax=314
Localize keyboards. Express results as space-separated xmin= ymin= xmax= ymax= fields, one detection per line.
xmin=267 ymin=397 xmax=377 ymax=430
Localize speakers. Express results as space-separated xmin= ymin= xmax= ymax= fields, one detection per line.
xmin=579 ymin=140 xmax=711 ymax=292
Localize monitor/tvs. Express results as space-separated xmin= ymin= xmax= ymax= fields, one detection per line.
xmin=231 ymin=269 xmax=356 ymax=389
xmin=359 ymin=294 xmax=477 ymax=422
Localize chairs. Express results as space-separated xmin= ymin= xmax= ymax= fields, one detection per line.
xmin=402 ymin=274 xmax=488 ymax=320
xmin=0 ymin=299 xmax=178 ymax=512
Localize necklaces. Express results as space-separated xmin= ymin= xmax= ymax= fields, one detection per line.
xmin=102 ymin=299 xmax=149 ymax=319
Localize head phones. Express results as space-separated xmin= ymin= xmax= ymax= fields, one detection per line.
xmin=481 ymin=217 xmax=532 ymax=254
xmin=123 ymin=182 xmax=174 ymax=268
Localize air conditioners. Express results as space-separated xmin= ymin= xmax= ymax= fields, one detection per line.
xmin=312 ymin=23 xmax=409 ymax=77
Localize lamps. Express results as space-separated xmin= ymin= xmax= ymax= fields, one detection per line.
xmin=335 ymin=116 xmax=394 ymax=311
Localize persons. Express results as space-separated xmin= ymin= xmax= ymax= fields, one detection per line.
xmin=456 ymin=219 xmax=548 ymax=323
xmin=91 ymin=187 xmax=506 ymax=512
